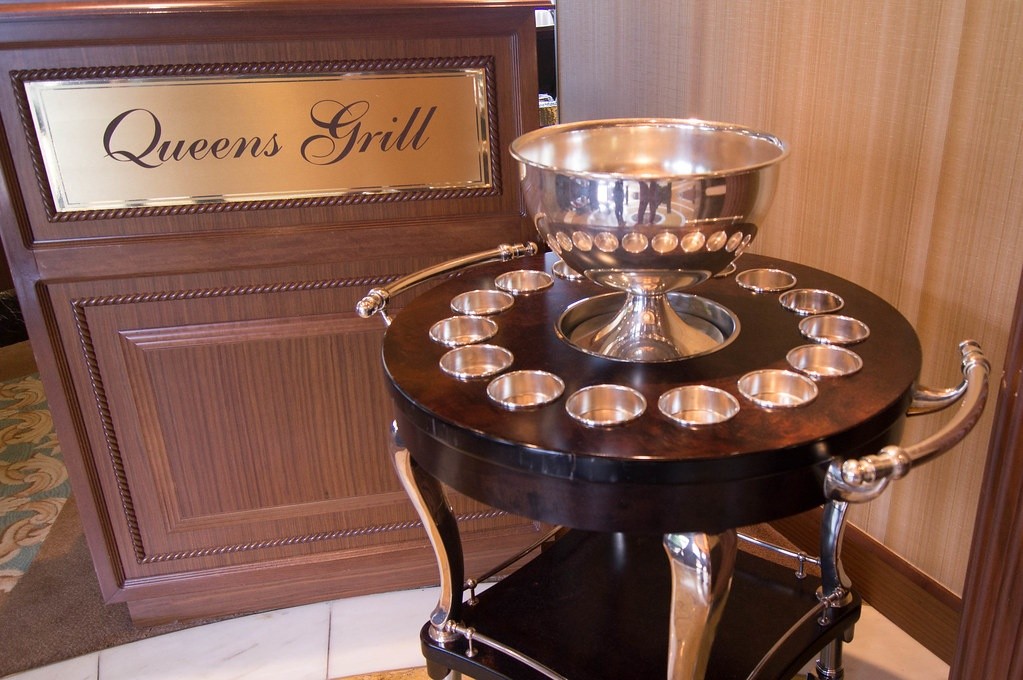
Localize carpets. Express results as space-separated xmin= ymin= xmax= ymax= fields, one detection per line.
xmin=1 ymin=336 xmax=293 ymax=677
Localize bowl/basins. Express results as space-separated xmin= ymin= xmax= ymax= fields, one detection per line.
xmin=657 ymin=262 xmax=869 ymax=425
xmin=546 ymin=227 xmax=752 ymax=256
xmin=430 ymin=260 xmax=647 ymax=425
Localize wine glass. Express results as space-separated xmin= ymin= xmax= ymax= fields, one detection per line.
xmin=508 ymin=116 xmax=793 ymax=361
